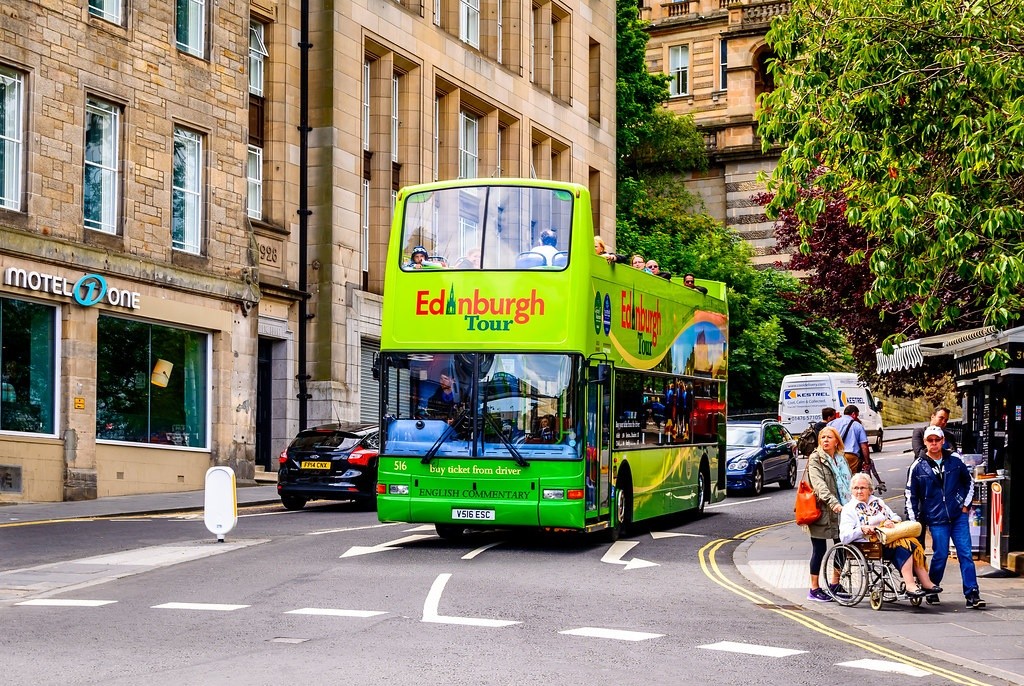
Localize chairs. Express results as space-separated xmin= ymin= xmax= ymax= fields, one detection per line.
xmin=419 ymin=381 xmax=438 ymax=420
xmin=529 ymin=413 xmax=570 ymax=444
xmin=515 ymin=251 xmax=568 ymax=269
xmin=428 ymin=256 xmax=467 ymax=267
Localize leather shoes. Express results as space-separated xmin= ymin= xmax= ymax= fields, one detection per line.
xmin=905 ymin=590 xmax=926 ymax=598
xmin=921 ymin=585 xmax=943 ymax=593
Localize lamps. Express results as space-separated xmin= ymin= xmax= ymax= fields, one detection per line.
xmin=151 ymin=360 xmax=174 ymax=388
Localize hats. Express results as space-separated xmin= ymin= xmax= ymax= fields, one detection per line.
xmin=924 ymin=426 xmax=944 ymax=440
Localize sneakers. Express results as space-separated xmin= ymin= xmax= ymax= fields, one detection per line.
xmin=925 ymin=593 xmax=941 ymax=605
xmin=966 ymin=596 xmax=986 ymax=609
xmin=807 ymin=588 xmax=834 ymax=602
xmin=828 ymin=584 xmax=852 ymax=600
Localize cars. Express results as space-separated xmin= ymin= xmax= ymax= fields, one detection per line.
xmin=276 ymin=421 xmax=381 ymax=512
xmin=723 ymin=419 xmax=799 ymax=495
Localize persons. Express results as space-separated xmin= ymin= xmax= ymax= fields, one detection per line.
xmin=903 ymin=425 xmax=987 ymax=609
xmin=911 ymin=406 xmax=957 ymax=578
xmin=682 ymin=273 xmax=708 ymax=294
xmin=422 ymin=369 xmax=463 ymax=426
xmin=838 ymin=473 xmax=943 ymax=598
xmin=631 ymin=255 xmax=653 ymax=275
xmin=645 ymin=261 xmax=671 ymax=280
xmin=593 ymin=237 xmax=626 ymax=265
xmin=646 ymin=378 xmax=695 ymax=440
xmin=530 ymin=417 xmax=552 ymax=440
xmin=806 ymin=427 xmax=854 ymax=603
xmin=827 ymin=406 xmax=871 ymax=475
xmin=812 ymin=408 xmax=837 ymax=436
xmin=459 ymin=247 xmax=481 ymax=270
xmin=405 ymin=246 xmax=446 ymax=269
xmin=530 ymin=229 xmax=563 ymax=265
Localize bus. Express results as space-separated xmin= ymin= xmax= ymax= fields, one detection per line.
xmin=371 ymin=177 xmax=730 ymax=543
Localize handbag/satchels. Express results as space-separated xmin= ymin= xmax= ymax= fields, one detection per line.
xmin=874 ymin=520 xmax=922 ymax=545
xmin=796 ymin=480 xmax=821 ymax=526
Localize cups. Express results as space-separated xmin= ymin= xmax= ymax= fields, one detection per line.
xmin=997 ymin=470 xmax=1004 ymax=475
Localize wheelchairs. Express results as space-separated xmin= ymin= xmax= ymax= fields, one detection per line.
xmin=822 ymin=508 xmax=939 ymax=613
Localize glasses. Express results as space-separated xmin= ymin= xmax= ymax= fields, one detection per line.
xmin=686 ymin=280 xmax=694 ymax=284
xmin=648 ymin=265 xmax=658 ymax=269
xmin=927 ymin=438 xmax=943 ymax=443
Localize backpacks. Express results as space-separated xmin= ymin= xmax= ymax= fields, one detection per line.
xmin=798 ymin=427 xmax=817 ymax=456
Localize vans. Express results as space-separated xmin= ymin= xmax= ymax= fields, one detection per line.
xmin=778 ymin=371 xmax=884 ymax=453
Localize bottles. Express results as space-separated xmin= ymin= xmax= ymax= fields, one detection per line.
xmin=974 ymin=466 xmax=984 ymax=478
